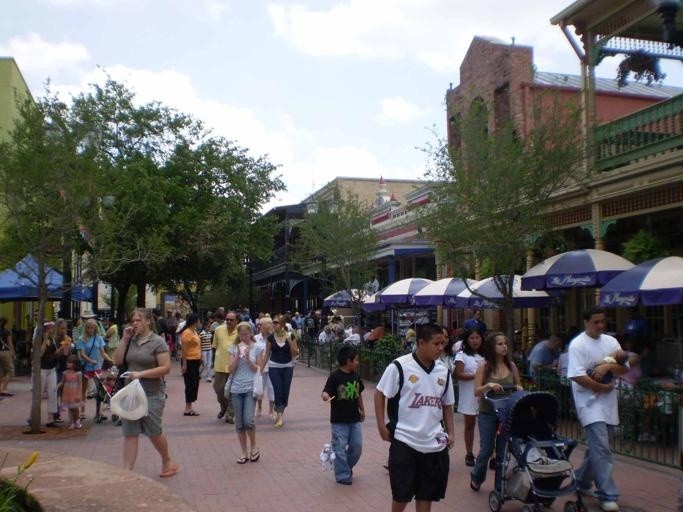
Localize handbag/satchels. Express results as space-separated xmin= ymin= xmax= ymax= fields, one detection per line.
xmin=102 ymin=366 xmax=128 ymax=404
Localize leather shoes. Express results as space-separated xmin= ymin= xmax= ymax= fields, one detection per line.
xmin=217 ymin=411 xmax=224 ymax=419
xmin=226 ymin=418 xmax=234 ymax=424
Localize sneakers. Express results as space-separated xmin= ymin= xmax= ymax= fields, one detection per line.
xmin=465 ymin=454 xmax=475 ymax=466
xmin=272 ymin=412 xmax=285 ymax=429
xmin=68 ymin=424 xmax=76 ymax=430
xmin=207 ymin=379 xmax=212 ymax=382
xmin=336 ymin=478 xmax=353 ymax=485
xmin=489 ymin=459 xmax=497 ymax=469
xmin=578 ymin=487 xmax=593 ymax=495
xmin=257 ymin=408 xmax=262 ymax=416
xmin=268 ymin=413 xmax=274 ymax=418
xmin=75 ymin=425 xmax=82 ymax=429
xmin=599 ymin=499 xmax=619 ymax=511
xmin=46 ymin=423 xmax=62 ymax=428
xmin=0 ymin=392 xmax=15 ymax=396
xmin=53 ymin=415 xmax=108 ymax=423
xmin=637 ymin=433 xmax=659 ymax=443
xmin=471 ymin=478 xmax=486 ymax=490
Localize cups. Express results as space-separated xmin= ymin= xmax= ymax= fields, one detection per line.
xmin=60 ymin=342 xmax=69 ymax=357
xmin=237 ymin=344 xmax=247 ymax=359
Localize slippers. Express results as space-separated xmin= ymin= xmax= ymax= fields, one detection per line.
xmin=160 ymin=464 xmax=180 ymax=477
xmin=237 ymin=457 xmax=249 ymax=464
xmin=184 ymin=410 xmax=200 ymax=416
xmin=250 ymin=450 xmax=260 ymax=461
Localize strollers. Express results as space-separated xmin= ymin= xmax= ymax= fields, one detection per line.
xmin=92 ymin=363 xmax=122 ymax=426
xmin=483 ymin=384 xmax=592 ymax=511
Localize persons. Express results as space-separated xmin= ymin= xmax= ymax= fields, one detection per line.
xmin=0 ymin=317 xmax=18 ymax=397
xmin=318 ymin=311 xmax=423 ymax=352
xmin=319 ymin=344 xmax=366 ymax=485
xmin=468 ymin=330 xmax=525 ymax=492
xmin=373 ymin=322 xmax=457 ymax=512
xmin=30 ymin=303 xmax=315 ymax=476
xmin=434 ymin=324 xmax=458 ymax=378
xmin=565 ymin=306 xmax=641 ymax=512
xmin=513 ymin=329 xmax=524 ymax=362
xmin=452 ymin=326 xmax=503 ymax=470
xmin=575 ymin=349 xmax=628 ymax=407
xmin=524 ymin=316 xmax=681 ymax=456
xmin=463 ymin=307 xmax=487 ymax=336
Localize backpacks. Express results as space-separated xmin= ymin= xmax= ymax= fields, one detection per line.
xmin=386 ymin=359 xmax=404 ymax=420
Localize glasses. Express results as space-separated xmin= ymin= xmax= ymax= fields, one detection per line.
xmin=226 ymin=318 xmax=237 ymax=321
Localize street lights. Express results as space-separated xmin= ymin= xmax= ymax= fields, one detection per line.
xmin=243 ymin=256 xmax=255 ymax=321
